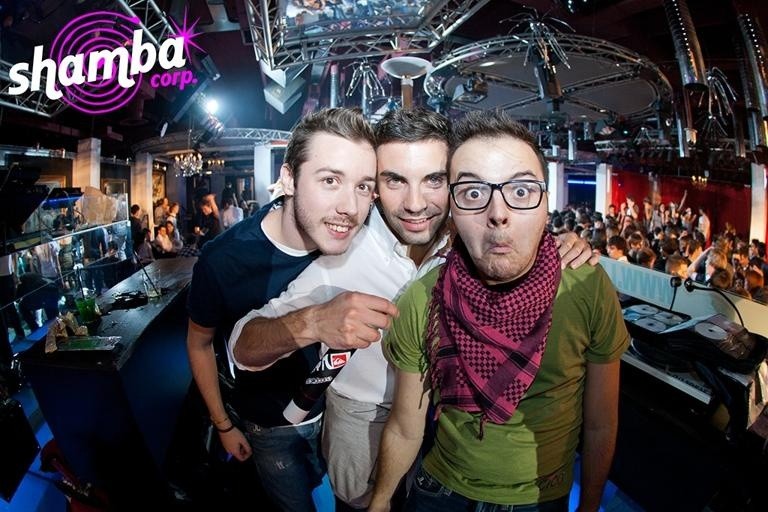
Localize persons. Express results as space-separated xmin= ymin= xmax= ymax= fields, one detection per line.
xmin=184 ymin=108 xmax=379 ymax=512
xmin=0 ymin=181 xmax=767 ymax=346
xmin=226 ymin=105 xmax=602 ymax=510
xmin=282 ymin=0 xmax=427 ymax=33
xmin=366 ymin=105 xmax=632 ymax=512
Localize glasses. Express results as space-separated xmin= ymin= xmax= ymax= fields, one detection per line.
xmin=449 ymin=180 xmax=548 ymax=210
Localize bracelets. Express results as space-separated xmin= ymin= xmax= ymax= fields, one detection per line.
xmin=209 ymin=412 xmax=228 ymax=425
xmin=214 ymin=423 xmax=236 ymax=433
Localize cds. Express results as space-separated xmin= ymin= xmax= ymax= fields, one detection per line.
xmin=630 ymin=304 xmax=659 ymax=315
xmin=635 ymin=318 xmax=666 ymax=333
xmin=654 ymin=311 xmax=684 ymax=326
xmin=695 ymin=322 xmax=728 ymax=340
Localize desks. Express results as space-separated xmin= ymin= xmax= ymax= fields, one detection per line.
xmin=19 ymin=257 xmax=199 ymax=486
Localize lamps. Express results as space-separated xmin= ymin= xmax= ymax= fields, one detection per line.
xmin=173 ymin=115 xmax=204 ymax=178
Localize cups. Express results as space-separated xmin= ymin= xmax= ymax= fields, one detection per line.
xmin=140 ymin=269 xmax=162 ymax=299
xmin=75 ymin=294 xmax=98 ymax=324
